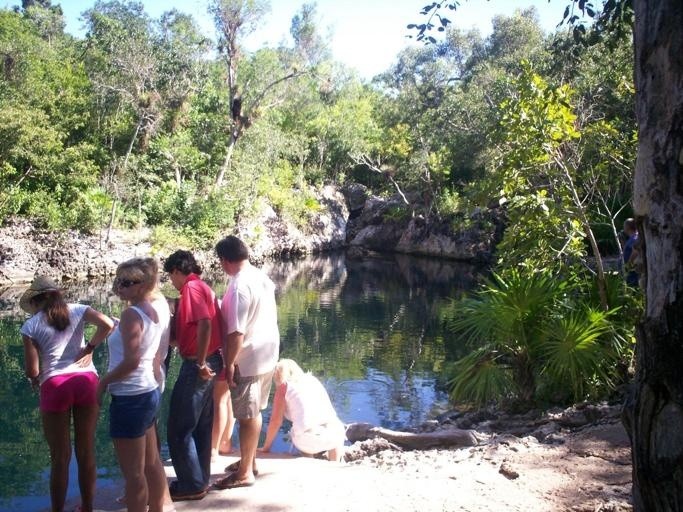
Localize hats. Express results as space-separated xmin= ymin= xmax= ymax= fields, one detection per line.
xmin=19 ymin=276 xmax=69 ymax=314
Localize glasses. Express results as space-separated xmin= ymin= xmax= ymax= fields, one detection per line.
xmin=116 ymin=277 xmax=145 ymax=289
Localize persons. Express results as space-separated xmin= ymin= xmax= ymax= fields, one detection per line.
xmin=213 ymin=237 xmax=280 ymax=489
xmin=115 ymin=258 xmax=170 ymax=506
xmin=19 ymin=276 xmax=114 ymax=512
xmin=95 ymin=258 xmax=168 ymax=512
xmin=163 ymin=250 xmax=224 ymax=500
xmin=256 ymin=358 xmax=346 ymax=462
xmin=211 ymin=299 xmax=237 ymax=458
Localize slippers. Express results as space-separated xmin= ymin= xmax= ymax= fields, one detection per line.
xmin=224 ymin=460 xmax=259 ymax=475
xmin=212 ymin=473 xmax=254 ymax=489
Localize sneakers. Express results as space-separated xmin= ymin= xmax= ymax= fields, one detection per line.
xmin=169 ymin=480 xmax=209 ymax=500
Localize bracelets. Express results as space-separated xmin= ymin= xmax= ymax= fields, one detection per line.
xmin=87 ymin=343 xmax=95 ymax=350
xmin=196 ymin=364 xmax=207 ymax=370
